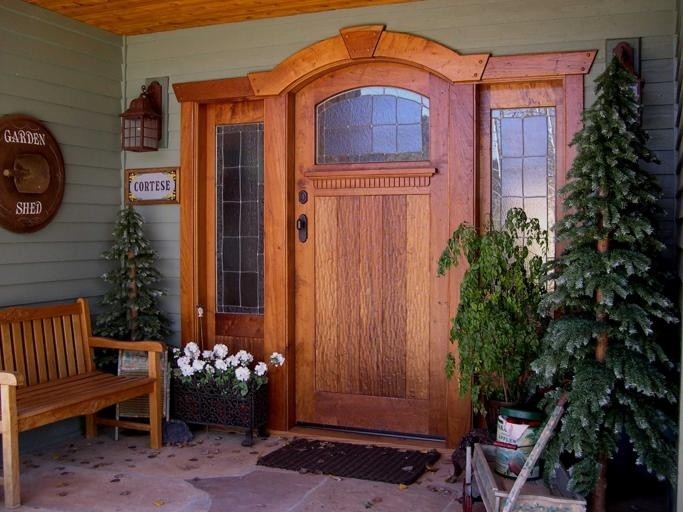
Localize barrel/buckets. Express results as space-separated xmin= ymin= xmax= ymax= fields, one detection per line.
xmin=491 ymin=404 xmax=548 ymax=482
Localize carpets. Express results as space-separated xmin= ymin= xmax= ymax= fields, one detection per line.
xmin=256 ymin=438 xmax=442 ymax=487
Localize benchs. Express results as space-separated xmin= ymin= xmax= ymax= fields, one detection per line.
xmin=0 ymin=298 xmax=167 ymax=508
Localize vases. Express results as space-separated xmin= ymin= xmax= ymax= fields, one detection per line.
xmin=170 ymin=378 xmax=270 ymax=448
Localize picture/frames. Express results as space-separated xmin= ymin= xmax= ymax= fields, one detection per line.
xmin=123 ymin=166 xmax=181 ymax=205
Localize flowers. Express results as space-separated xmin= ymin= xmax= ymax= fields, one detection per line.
xmin=168 ymin=340 xmax=285 ymax=398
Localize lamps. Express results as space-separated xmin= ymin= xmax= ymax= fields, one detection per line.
xmin=116 ymin=80 xmax=163 ymax=153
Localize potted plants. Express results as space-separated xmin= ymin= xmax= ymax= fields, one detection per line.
xmin=437 ymin=207 xmax=559 ymax=447
